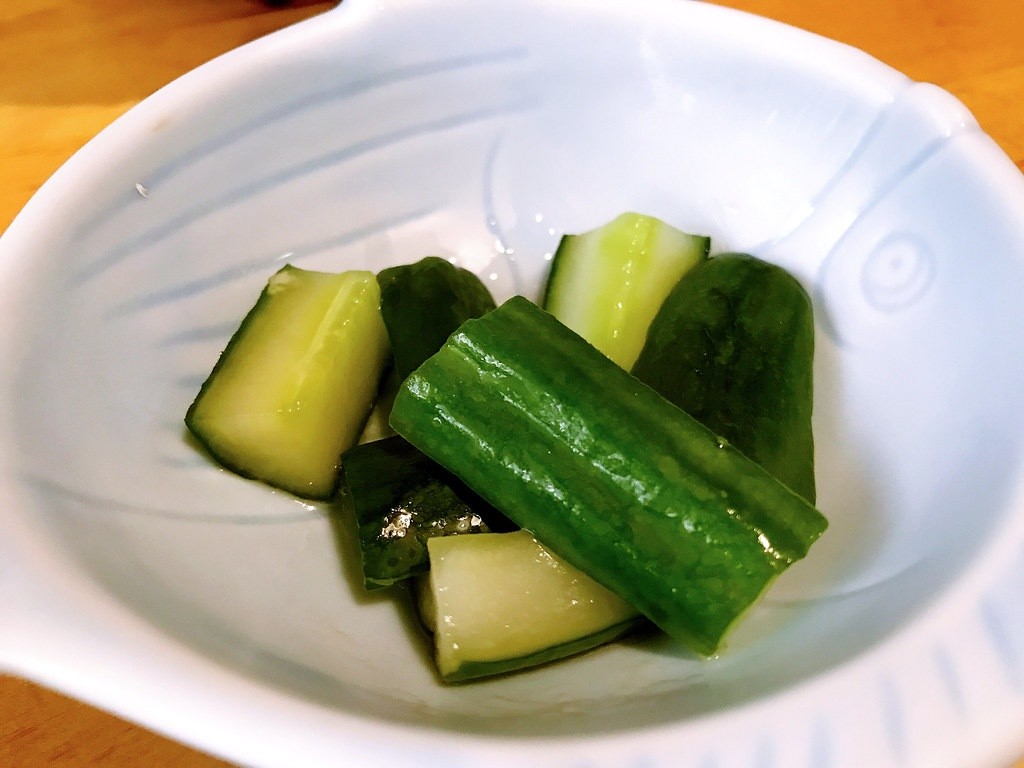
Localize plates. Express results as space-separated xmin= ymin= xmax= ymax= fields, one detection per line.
xmin=0 ymin=0 xmax=1024 ymax=768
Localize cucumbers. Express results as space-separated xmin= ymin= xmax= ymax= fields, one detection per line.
xmin=184 ymin=212 xmax=831 ymax=687
